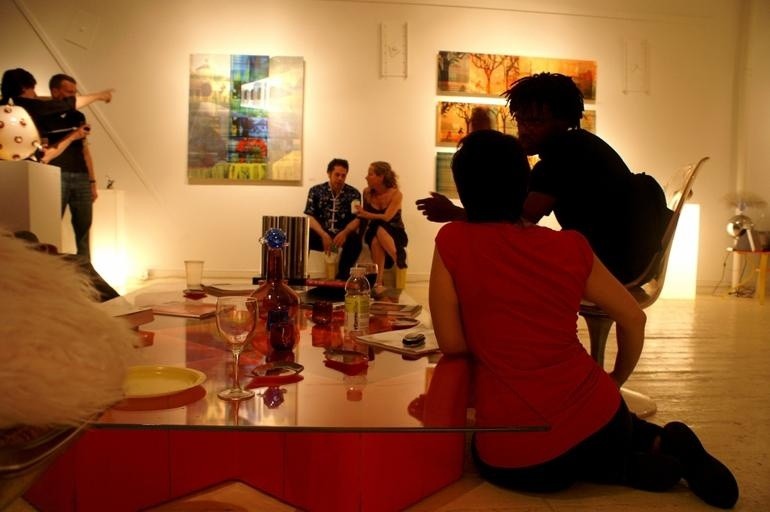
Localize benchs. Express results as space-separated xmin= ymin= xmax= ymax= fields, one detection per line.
xmin=308 ymin=242 xmax=407 ymax=289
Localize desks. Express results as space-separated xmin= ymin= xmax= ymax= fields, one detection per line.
xmin=724 ymin=245 xmax=770 ymax=306
xmin=21 ymin=275 xmax=551 ymax=510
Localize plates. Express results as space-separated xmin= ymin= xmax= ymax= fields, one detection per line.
xmin=323 ymin=347 xmax=368 ymax=367
xmin=244 ymin=375 xmax=304 ymax=390
xmin=123 ymin=365 xmax=207 ymax=400
xmin=114 ymin=386 xmax=205 ymax=409
xmin=245 ymin=361 xmax=302 ymax=378
xmin=321 ymin=361 xmax=366 ymax=377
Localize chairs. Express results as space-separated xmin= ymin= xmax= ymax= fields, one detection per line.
xmin=579 ymin=156 xmax=714 ymax=421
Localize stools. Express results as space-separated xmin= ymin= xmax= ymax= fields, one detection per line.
xmin=0 ymin=403 xmax=113 ymax=512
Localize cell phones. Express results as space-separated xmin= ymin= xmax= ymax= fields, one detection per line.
xmin=402 ymin=333 xmax=426 ymax=347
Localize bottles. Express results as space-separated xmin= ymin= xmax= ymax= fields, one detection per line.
xmin=343 ymin=267 xmax=370 ymax=330
xmin=247 ymin=247 xmax=301 ymax=358
xmin=341 ymin=370 xmax=368 ymax=402
xmin=350 ymin=199 xmax=360 ymax=214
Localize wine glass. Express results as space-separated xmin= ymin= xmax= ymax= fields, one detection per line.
xmin=214 ymin=295 xmax=255 ymax=400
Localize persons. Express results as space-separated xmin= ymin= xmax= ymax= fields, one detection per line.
xmin=410 ymin=70 xmax=667 ymax=285
xmin=40 ymin=74 xmax=98 ymax=270
xmin=303 ymin=157 xmax=361 ymax=290
xmin=0 ymin=68 xmax=114 ymax=163
xmin=429 ymin=127 xmax=739 ymax=509
xmin=354 ymin=160 xmax=409 ymax=295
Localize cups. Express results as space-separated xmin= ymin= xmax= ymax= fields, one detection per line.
xmin=184 ymin=260 xmax=204 ymax=289
xmin=326 ymin=262 xmax=336 ymax=280
xmin=356 ymin=262 xmax=378 ymax=289
xmin=312 ymin=302 xmax=333 ymax=324
xmin=312 ymin=325 xmax=332 ymax=348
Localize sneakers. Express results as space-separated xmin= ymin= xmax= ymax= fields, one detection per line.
xmin=656 ymin=433 xmax=739 ymax=508
